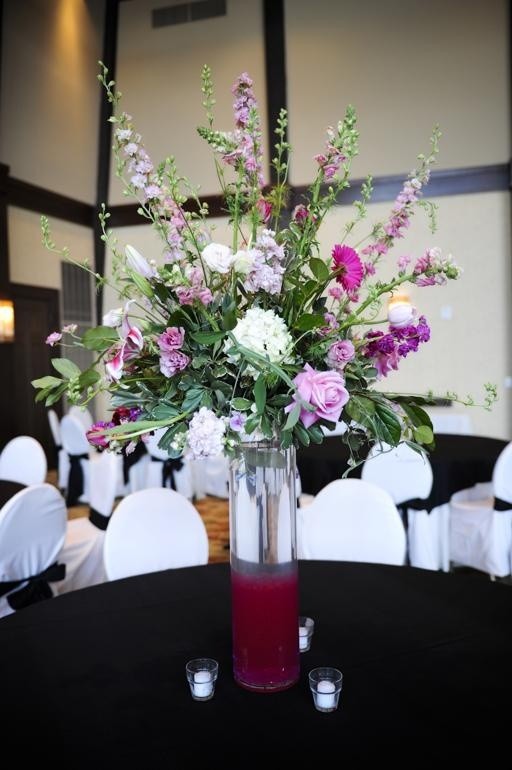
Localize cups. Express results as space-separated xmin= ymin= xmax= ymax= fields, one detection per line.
xmin=296 ymin=615 xmax=315 ymax=653
xmin=308 ymin=667 xmax=343 ymax=711
xmin=185 ymin=658 xmax=218 ymax=701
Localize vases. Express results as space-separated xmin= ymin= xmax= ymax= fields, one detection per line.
xmin=223 ymin=434 xmax=303 ymax=693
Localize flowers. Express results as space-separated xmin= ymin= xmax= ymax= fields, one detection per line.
xmin=20 ymin=55 xmax=501 ymax=478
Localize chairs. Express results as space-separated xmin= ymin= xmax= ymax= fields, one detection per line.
xmin=100 ymin=485 xmax=211 ymax=579
xmin=450 ymin=437 xmax=512 ymax=585
xmin=0 ymin=431 xmax=68 ymax=608
xmin=48 ymin=401 xmax=251 ymax=531
xmin=302 ymin=475 xmax=407 ymax=563
xmin=362 ymin=431 xmax=435 ymax=510
